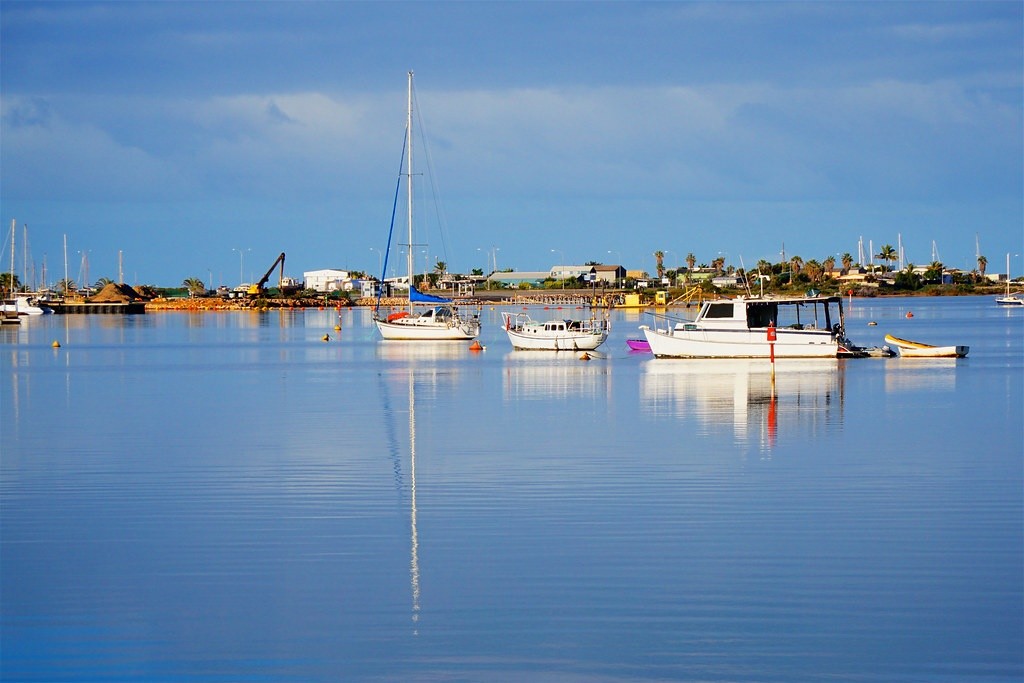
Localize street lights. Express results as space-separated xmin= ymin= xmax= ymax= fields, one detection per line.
xmin=551 ymin=248 xmax=564 ymax=291
xmin=476 ymin=248 xmax=500 ymax=292
xmin=665 ymin=250 xmax=678 ymax=289
xmin=369 ymin=247 xmax=391 ymax=281
xmin=607 ymin=250 xmax=621 ymax=289
xmin=233 ymin=247 xmax=251 ymax=285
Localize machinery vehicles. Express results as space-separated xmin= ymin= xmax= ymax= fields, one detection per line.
xmin=244 ymin=252 xmax=286 ymax=300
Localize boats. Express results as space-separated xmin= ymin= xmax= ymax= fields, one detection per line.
xmin=502 ymin=349 xmax=608 ymax=361
xmin=884 ymin=333 xmax=970 ymax=358
xmin=637 ymin=294 xmax=896 ymax=359
xmin=886 ymin=356 xmax=970 ymax=370
xmin=627 ymin=338 xmax=651 ymax=350
xmin=500 ymin=310 xmax=610 ymax=350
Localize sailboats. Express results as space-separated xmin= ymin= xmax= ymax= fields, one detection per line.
xmin=372 ymin=68 xmax=483 ymax=342
xmin=0 ymin=219 xmax=56 ymax=325
xmin=995 ymin=253 xmax=1024 ymax=305
xmin=375 ymin=340 xmax=480 ymax=621
